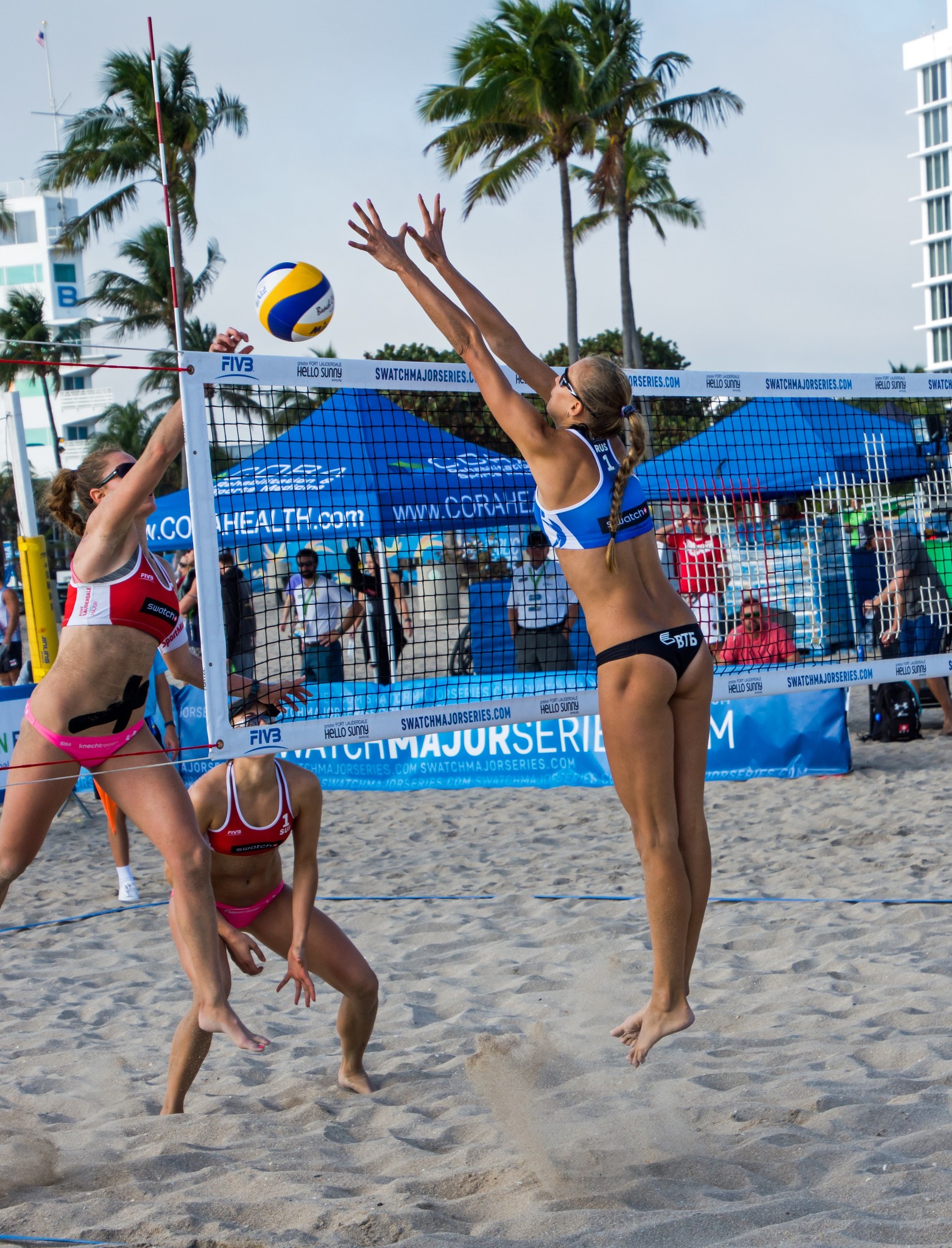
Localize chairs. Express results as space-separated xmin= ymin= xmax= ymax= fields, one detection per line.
xmin=719 ymin=607 xmax=796 ymax=642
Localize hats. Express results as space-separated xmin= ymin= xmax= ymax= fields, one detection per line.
xmin=855 ymin=519 xmax=886 ymax=549
xmin=229 ymin=698 xmax=281 ymax=722
xmin=527 ymin=532 xmax=548 ymax=545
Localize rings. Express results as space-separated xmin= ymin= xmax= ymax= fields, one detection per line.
xmin=326 ymin=638 xmax=329 ymax=641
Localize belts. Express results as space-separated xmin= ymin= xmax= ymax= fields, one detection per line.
xmin=522 ymin=622 xmax=564 ymax=633
xmin=306 ymin=641 xmax=320 ymax=647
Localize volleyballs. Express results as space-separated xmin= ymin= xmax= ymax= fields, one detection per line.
xmin=252 ymin=260 xmax=334 ymax=342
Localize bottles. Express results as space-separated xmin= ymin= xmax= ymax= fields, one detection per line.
xmin=347 ymin=638 xmax=354 ymax=657
xmin=874 ymin=713 xmax=880 ymax=727
xmin=865 ymin=595 xmax=878 ymax=619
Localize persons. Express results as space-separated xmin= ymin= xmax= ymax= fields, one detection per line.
xmin=655 ymin=500 xmax=729 ymax=657
xmin=780 ymin=496 xmax=811 ymax=520
xmin=159 ymin=697 xmax=379 ymax=1117
xmin=0 ymin=559 xmax=23 ymax=687
xmin=507 ymin=530 xmax=579 ymax=672
xmin=825 ymin=497 xmax=850 ymax=529
xmin=0 ymin=327 xmax=270 ymax=1054
xmin=348 ymin=192 xmax=715 ymax=1069
xmin=844 ymin=494 xmax=873 ymax=560
xmin=854 ymin=521 xmax=952 ymax=738
xmin=719 ymin=597 xmax=801 ymax=667
xmin=93 ymin=647 xmax=179 ymax=902
xmin=169 ymin=548 xmax=415 ymax=685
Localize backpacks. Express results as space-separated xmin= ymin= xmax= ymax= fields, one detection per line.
xmin=869 ymin=681 xmax=922 ymax=743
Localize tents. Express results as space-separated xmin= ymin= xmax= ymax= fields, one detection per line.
xmin=149 ymin=387 xmax=538 ymax=688
xmin=632 ymin=397 xmax=949 ymax=663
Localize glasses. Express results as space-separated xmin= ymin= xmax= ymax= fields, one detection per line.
xmin=89 ymin=463 xmax=135 ymax=504
xmin=179 ymin=562 xmax=187 ymax=567
xmin=688 ymin=520 xmax=707 ymax=525
xmin=298 ymin=560 xmax=313 ymax=567
xmin=232 ymin=714 xmax=277 ymax=729
xmin=529 ymin=544 xmax=545 ymax=550
xmin=740 ymin=611 xmax=760 ymax=618
xmin=559 ymin=367 xmax=599 ymax=419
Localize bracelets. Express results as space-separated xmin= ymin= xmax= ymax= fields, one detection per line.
xmin=562 ymin=627 xmax=572 ymax=633
xmin=248 ymin=679 xmax=260 ymax=700
xmin=675 ymin=521 xmax=679 ymax=529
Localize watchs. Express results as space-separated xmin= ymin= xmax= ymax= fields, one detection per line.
xmin=164 ymin=719 xmax=176 ymax=729
xmin=404 ymin=618 xmax=412 ymax=622
xmin=337 ymin=628 xmax=345 ymax=635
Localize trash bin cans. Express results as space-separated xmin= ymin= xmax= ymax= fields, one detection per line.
xmin=416 ymin=563 xmax=460 ymax=620
